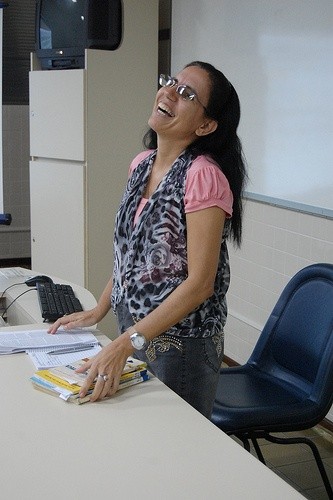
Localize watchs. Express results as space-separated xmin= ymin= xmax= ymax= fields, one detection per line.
xmin=125 ymin=326 xmax=148 ymax=351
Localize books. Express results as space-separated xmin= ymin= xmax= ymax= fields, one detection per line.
xmin=0 ymin=327 xmax=150 ymax=404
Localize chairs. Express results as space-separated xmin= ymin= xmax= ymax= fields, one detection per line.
xmin=210 ymin=263 xmax=333 ymax=500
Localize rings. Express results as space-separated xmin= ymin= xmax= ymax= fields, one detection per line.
xmin=97 ymin=374 xmax=108 ymax=382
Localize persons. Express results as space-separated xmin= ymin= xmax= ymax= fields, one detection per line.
xmin=47 ymin=62 xmax=250 ymax=419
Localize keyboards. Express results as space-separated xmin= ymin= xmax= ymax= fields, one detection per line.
xmin=35 ymin=281 xmax=82 ymax=322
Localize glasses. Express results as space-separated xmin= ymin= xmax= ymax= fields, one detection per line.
xmin=159 ymin=74 xmax=214 ymax=119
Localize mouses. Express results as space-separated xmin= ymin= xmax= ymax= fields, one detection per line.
xmin=25 ymin=275 xmax=53 ymax=287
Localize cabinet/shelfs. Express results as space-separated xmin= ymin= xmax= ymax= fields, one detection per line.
xmin=28 ymin=0 xmax=159 ymax=340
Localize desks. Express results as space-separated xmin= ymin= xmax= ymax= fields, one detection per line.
xmin=0 ymin=267 xmax=99 ymax=331
xmin=0 ymin=321 xmax=307 ymax=500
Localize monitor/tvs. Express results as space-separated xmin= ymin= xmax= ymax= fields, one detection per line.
xmin=35 ymin=0 xmax=121 ymax=71
